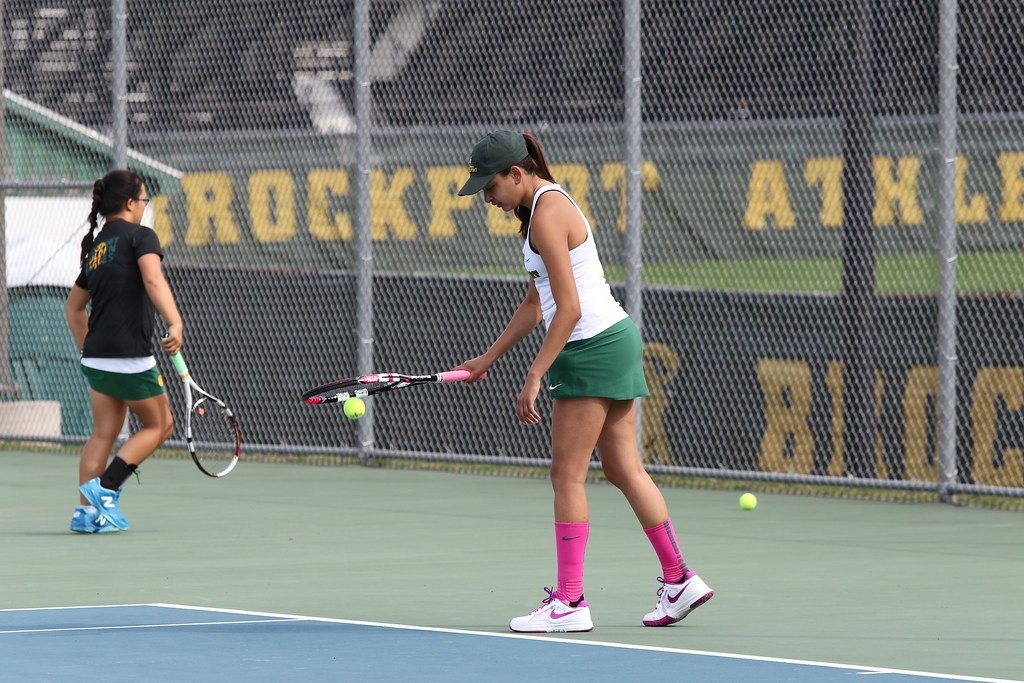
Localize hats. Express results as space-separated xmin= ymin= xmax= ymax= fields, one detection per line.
xmin=457 ymin=130 xmax=529 ymax=197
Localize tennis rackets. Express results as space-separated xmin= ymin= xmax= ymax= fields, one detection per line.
xmin=304 ymin=367 xmax=490 ymax=406
xmin=163 ymin=332 xmax=242 ymax=477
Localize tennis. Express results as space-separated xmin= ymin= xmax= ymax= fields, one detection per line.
xmin=342 ymin=397 xmax=366 ymax=420
xmin=739 ymin=493 xmax=757 ymax=511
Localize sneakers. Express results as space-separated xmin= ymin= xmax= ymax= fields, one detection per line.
xmin=642 ymin=572 xmax=714 ymax=626
xmin=70 ymin=505 xmax=121 ymax=532
xmin=79 ymin=477 xmax=128 ymax=531
xmin=509 ymin=586 xmax=593 ymax=632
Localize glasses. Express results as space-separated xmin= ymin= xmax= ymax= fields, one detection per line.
xmin=128 ymin=197 xmax=149 ymax=206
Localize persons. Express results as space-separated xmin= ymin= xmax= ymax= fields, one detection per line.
xmin=65 ymin=171 xmax=184 ymax=535
xmin=449 ymin=130 xmax=714 ymax=634
